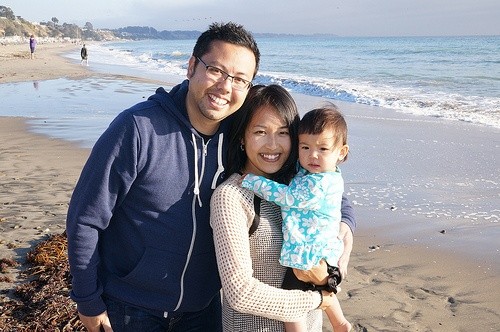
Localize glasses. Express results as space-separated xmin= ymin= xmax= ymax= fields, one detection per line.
xmin=197 ymin=57 xmax=252 ymax=92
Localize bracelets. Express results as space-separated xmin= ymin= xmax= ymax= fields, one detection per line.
xmin=314 ymin=290 xmax=323 ymax=311
xmin=313 ymin=266 xmax=343 ymax=294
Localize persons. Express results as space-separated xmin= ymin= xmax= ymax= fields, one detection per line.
xmin=29 ymin=35 xmax=36 ymax=60
xmin=239 ymin=102 xmax=356 ymax=332
xmin=65 ymin=21 xmax=356 ymax=332
xmin=80 ymin=43 xmax=89 ymax=66
xmin=208 ymin=84 xmax=345 ymax=332
